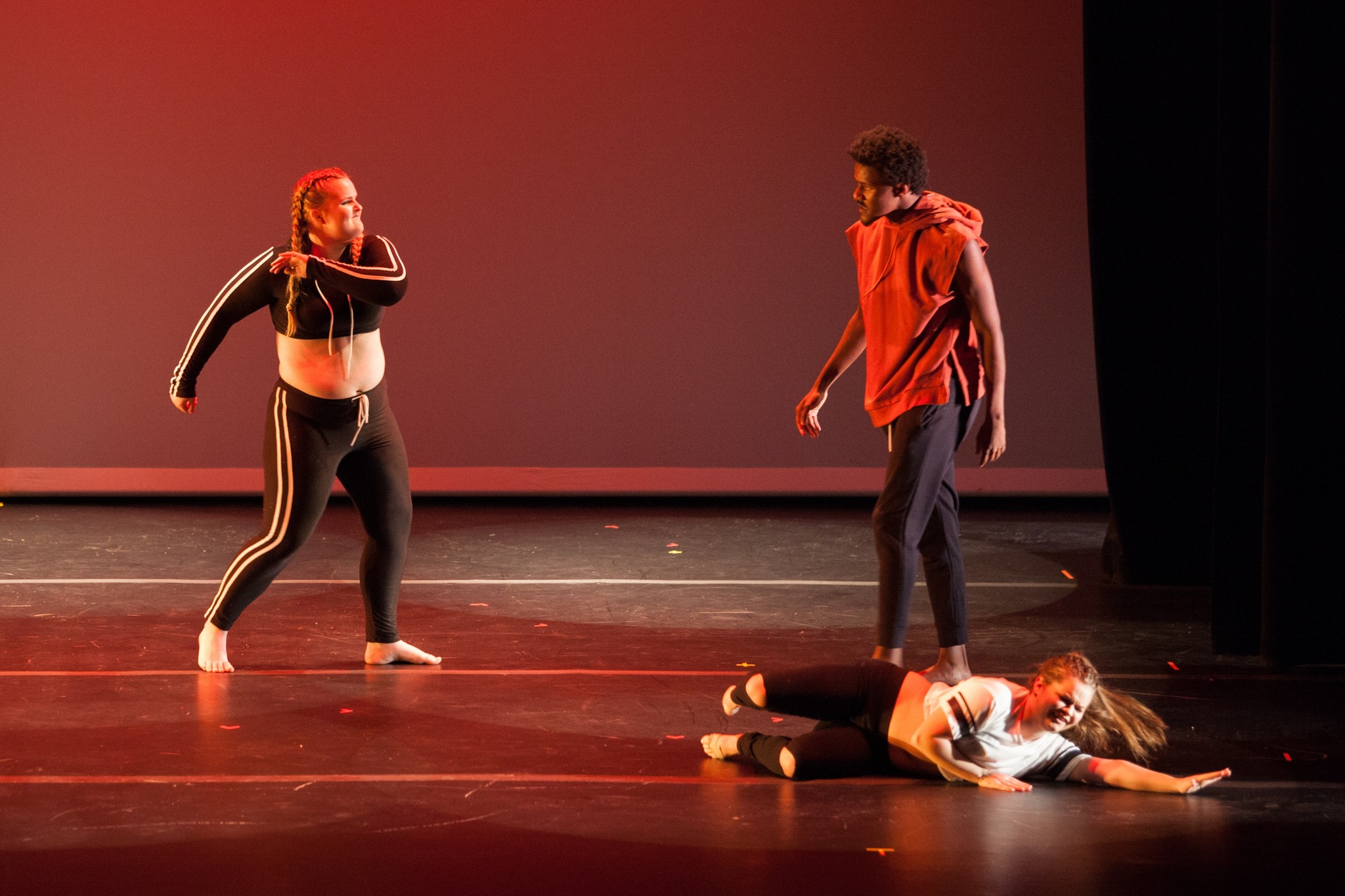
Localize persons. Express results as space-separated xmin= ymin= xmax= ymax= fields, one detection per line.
xmin=795 ymin=130 xmax=1005 ymax=685
xmin=701 ymin=651 xmax=1231 ymax=794
xmin=168 ymin=166 xmax=442 ymax=673
